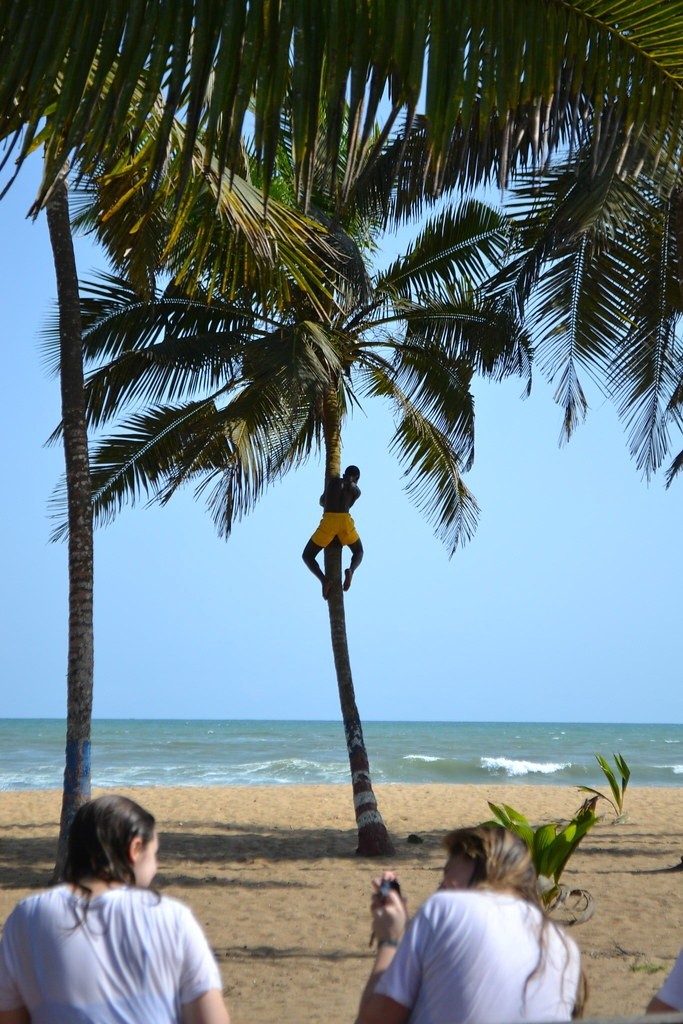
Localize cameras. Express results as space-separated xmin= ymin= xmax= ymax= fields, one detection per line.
xmin=379 ymin=879 xmax=401 ymax=903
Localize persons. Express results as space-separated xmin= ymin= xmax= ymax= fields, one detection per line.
xmin=646 ymin=946 xmax=683 ymax=1014
xmin=0 ymin=794 xmax=233 ymax=1024
xmin=354 ymin=822 xmax=581 ymax=1024
xmin=302 ymin=465 xmax=364 ymax=601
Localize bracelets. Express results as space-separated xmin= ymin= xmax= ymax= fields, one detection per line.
xmin=377 ymin=938 xmax=399 ymax=948
